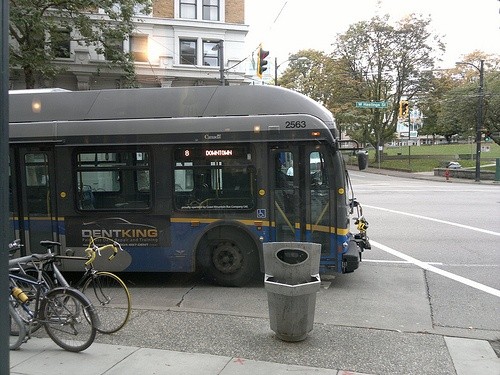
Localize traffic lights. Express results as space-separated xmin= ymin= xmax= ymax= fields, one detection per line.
xmin=257 ymin=45 xmax=269 ymax=78
xmin=400 ymin=101 xmax=408 ymax=118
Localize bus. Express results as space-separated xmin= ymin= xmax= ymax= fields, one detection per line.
xmin=9 ymin=86 xmax=371 ymax=287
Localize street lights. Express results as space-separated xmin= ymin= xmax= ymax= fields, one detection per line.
xmin=275 ymin=57 xmax=307 ymax=86
xmin=455 ymin=58 xmax=484 ymax=181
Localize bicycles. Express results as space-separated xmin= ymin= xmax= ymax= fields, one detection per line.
xmin=7 ymin=236 xmax=130 ymax=352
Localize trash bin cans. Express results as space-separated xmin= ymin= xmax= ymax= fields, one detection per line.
xmin=357 ymin=154 xmax=368 ymax=171
xmin=263 ymin=241 xmax=322 ymax=342
